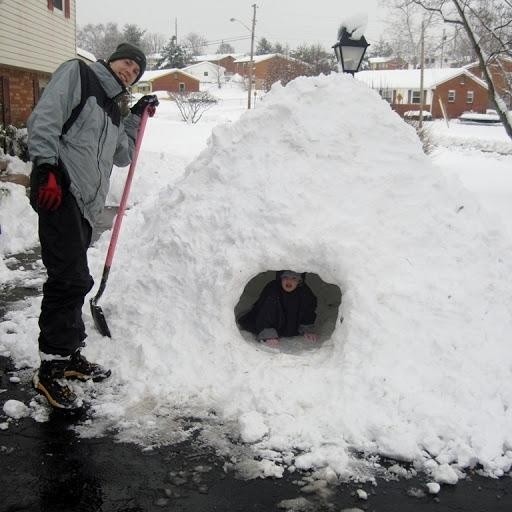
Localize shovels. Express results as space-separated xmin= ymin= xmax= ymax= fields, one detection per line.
xmin=89 ymin=102 xmax=151 ymax=338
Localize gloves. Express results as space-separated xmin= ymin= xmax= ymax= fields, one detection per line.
xmin=129 ymin=94 xmax=159 ymax=117
xmin=35 ymin=162 xmax=62 ymax=212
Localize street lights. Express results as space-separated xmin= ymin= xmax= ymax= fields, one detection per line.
xmin=230 ymin=4 xmax=259 ymax=109
xmin=332 ymin=19 xmax=371 ymax=79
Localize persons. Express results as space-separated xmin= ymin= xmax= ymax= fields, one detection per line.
xmin=23 ymin=41 xmax=160 ymax=410
xmin=238 ymin=270 xmax=318 ymax=342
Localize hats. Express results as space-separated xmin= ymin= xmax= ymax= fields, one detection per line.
xmin=108 ymin=44 xmax=146 ymax=86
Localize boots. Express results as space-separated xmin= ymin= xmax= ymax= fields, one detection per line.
xmin=33 ymin=350 xmax=81 ymax=410
xmin=65 ymin=342 xmax=111 ymax=383
